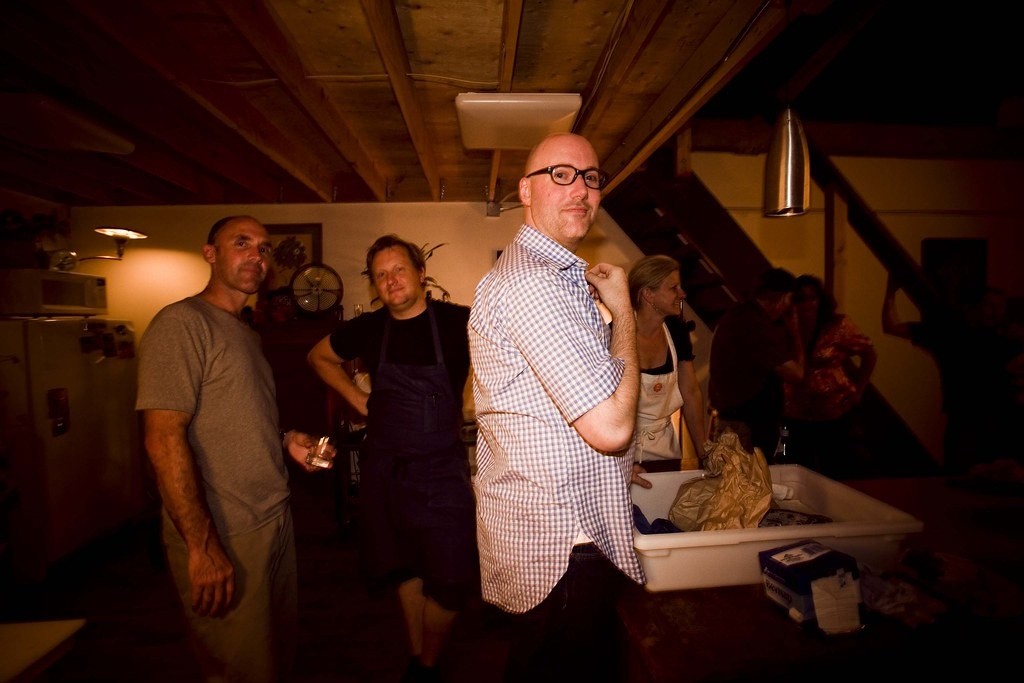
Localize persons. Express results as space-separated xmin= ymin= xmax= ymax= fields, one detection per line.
xmin=882 ymin=266 xmax=1024 ymax=477
xmin=467 ymin=133 xmax=652 ymax=683
xmin=307 ymin=237 xmax=470 ymax=683
xmin=709 ymin=268 xmax=877 ymax=479
xmin=137 ymin=216 xmax=336 ymax=683
xmin=607 ymin=255 xmax=708 ymax=473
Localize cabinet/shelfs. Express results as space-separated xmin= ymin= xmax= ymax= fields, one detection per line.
xmin=258 ymin=321 xmax=355 ymax=540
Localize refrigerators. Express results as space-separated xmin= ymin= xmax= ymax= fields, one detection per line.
xmin=1 ymin=313 xmax=139 ymax=583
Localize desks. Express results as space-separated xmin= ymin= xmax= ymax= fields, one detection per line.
xmin=0 ymin=619 xmax=88 ymax=683
xmin=612 ymin=476 xmax=1024 ymax=683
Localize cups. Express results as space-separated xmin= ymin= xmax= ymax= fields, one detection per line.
xmin=353 ymin=304 xmax=364 ymax=318
xmin=304 ymin=431 xmax=339 ymax=469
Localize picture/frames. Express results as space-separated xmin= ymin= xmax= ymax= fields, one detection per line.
xmin=257 ymin=222 xmax=323 ymax=303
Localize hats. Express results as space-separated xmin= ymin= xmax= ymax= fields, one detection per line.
xmin=756 ymin=268 xmax=808 ymax=305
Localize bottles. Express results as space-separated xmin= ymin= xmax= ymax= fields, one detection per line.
xmin=770 ymin=424 xmax=796 ymax=465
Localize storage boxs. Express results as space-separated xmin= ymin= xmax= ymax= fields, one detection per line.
xmin=631 ymin=465 xmax=922 ymax=592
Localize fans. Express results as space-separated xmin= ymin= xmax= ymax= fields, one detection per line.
xmin=289 ymin=262 xmax=344 ymax=322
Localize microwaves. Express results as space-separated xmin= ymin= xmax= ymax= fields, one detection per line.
xmin=1 ymin=268 xmax=109 ymax=315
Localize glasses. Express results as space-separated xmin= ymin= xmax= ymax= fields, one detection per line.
xmin=525 ymin=164 xmax=610 ymax=189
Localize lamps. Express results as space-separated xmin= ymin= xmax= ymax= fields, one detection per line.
xmin=62 ymin=225 xmax=150 ymax=265
xmin=454 ymin=90 xmax=583 ymax=150
xmin=0 ymin=92 xmax=136 ymax=154
xmin=763 ymin=26 xmax=811 ymax=218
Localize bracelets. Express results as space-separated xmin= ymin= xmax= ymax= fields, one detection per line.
xmin=279 ymin=432 xmax=284 ymax=440
xmin=700 ymin=452 xmax=710 ymax=459
